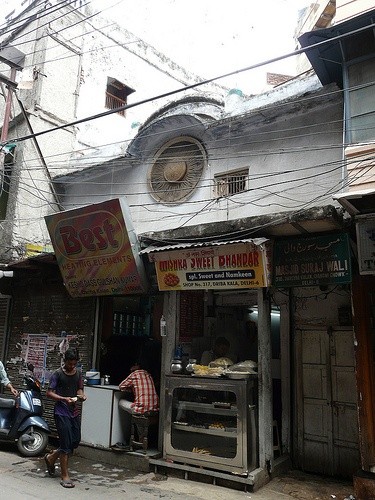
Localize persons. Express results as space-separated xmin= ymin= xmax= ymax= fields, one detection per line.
xmin=44 ymin=347 xmax=87 ymax=488
xmin=0 ymin=360 xmax=19 ymax=397
xmin=118 ymin=359 xmax=160 ymax=447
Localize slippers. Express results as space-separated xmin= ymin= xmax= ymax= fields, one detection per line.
xmin=45 ymin=454 xmax=55 ymax=477
xmin=60 ymin=479 xmax=75 ymax=488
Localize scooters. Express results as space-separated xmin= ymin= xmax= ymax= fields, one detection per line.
xmin=0 ymin=363 xmax=51 ymax=458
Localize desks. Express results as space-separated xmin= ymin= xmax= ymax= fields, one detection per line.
xmin=81 ymin=384 xmax=135 ymax=448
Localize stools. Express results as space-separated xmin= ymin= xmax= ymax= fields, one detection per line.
xmin=130 ymin=415 xmax=159 ymax=454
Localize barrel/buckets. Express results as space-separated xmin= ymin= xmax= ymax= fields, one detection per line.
xmin=84 ymin=369 xmax=100 ymax=386
